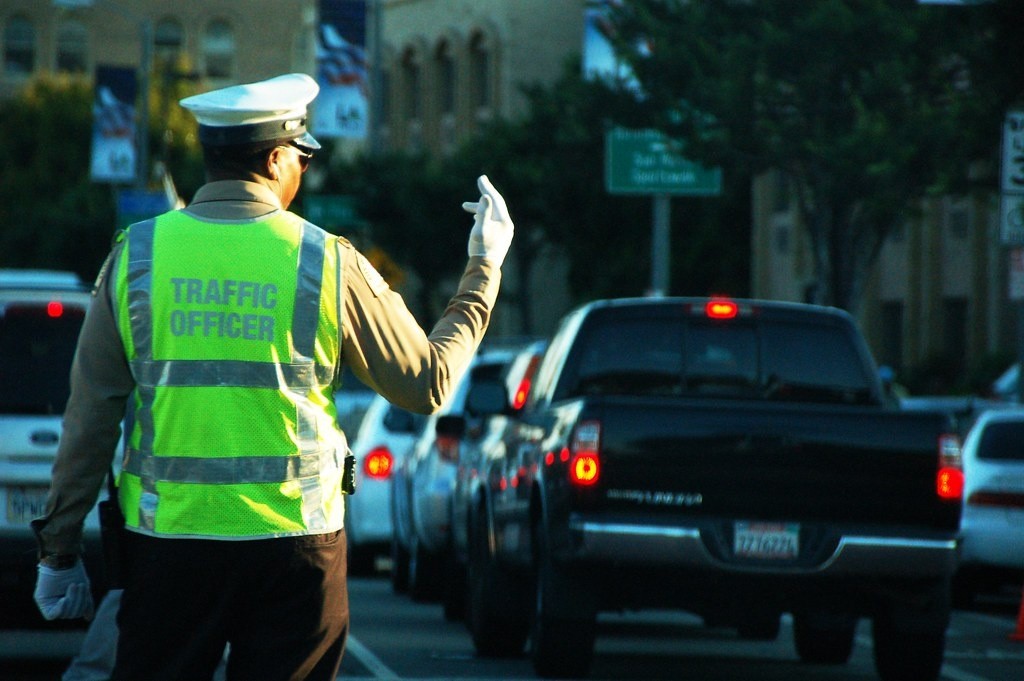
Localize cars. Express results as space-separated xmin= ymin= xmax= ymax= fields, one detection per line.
xmin=895 ymin=394 xmax=1024 ymax=617
xmin=434 ymin=341 xmax=552 ymax=660
xmin=383 ymin=352 xmax=517 ymax=605
xmin=347 ymin=393 xmax=430 ymax=582
xmin=332 ymin=388 xmax=377 ymax=443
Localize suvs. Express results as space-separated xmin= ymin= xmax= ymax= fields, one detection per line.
xmin=0 ymin=269 xmax=123 ymax=610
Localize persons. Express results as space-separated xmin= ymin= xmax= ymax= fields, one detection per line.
xmin=32 ymin=72 xmax=514 ymax=679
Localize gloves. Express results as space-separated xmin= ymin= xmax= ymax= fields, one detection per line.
xmin=462 ymin=175 xmax=515 ymax=268
xmin=33 ymin=559 xmax=94 ymax=621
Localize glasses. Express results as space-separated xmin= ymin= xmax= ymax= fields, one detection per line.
xmin=274 ymin=145 xmax=313 ymax=173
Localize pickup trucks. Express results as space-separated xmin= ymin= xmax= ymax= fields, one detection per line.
xmin=465 ymin=296 xmax=963 ymax=679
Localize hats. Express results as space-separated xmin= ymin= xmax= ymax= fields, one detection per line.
xmin=178 ymin=72 xmax=323 ymax=150
